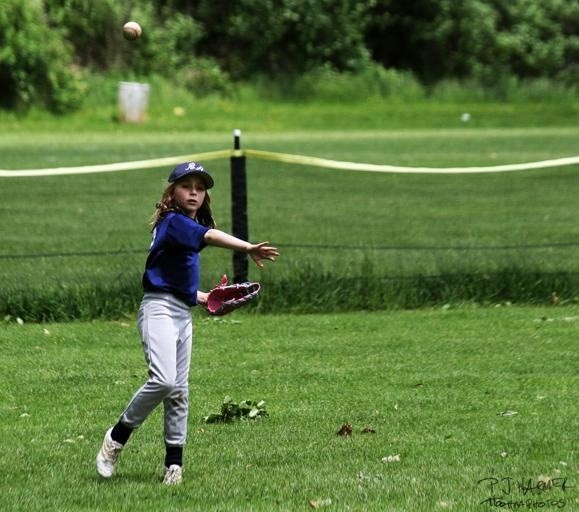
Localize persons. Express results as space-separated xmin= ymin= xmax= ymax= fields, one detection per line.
xmin=97 ymin=163 xmax=280 ymax=486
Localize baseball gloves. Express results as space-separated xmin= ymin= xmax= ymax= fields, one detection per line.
xmin=206 ymin=274 xmax=260 ymax=315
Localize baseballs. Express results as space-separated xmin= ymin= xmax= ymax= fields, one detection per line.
xmin=123 ymin=21 xmax=142 ymax=40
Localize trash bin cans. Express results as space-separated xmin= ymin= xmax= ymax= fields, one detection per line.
xmin=118 ymin=81 xmax=149 ymax=125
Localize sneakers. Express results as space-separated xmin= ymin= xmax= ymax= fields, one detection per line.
xmin=161 ymin=464 xmax=182 ymax=489
xmin=94 ymin=427 xmax=123 ymax=479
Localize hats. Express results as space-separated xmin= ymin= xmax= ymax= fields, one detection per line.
xmin=168 ymin=162 xmax=214 ymax=188
